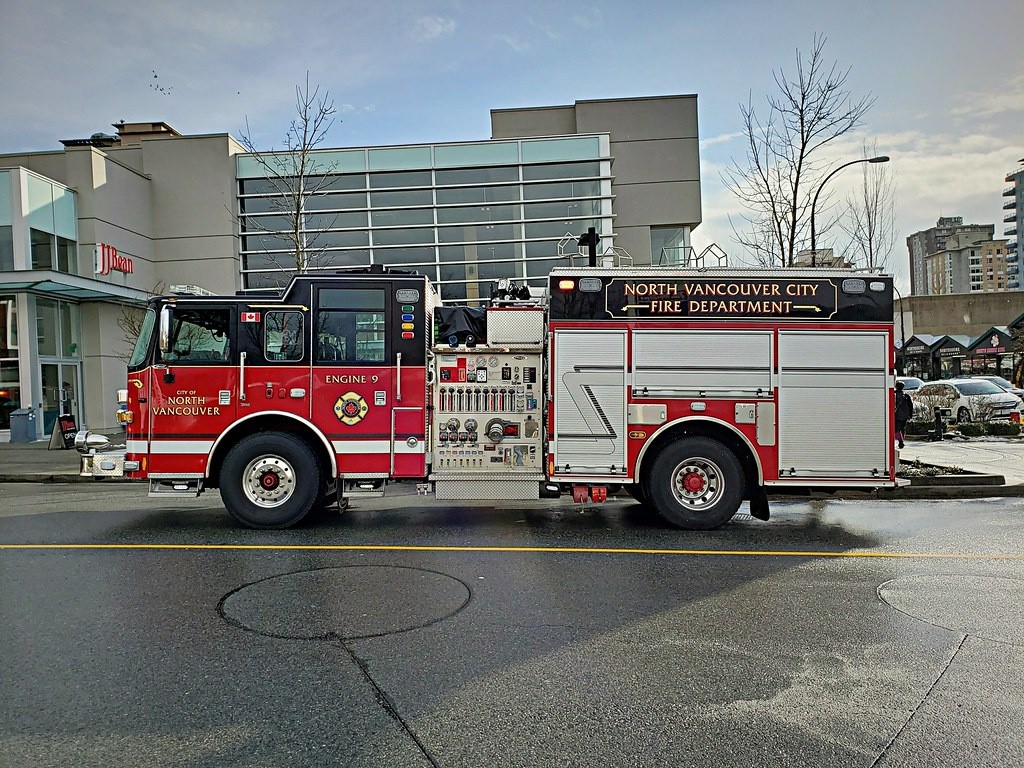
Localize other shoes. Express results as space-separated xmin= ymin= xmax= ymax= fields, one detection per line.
xmin=899 ymin=440 xmax=905 ymax=448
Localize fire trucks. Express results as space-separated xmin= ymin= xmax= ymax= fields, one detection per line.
xmin=73 ymin=264 xmax=911 ymax=530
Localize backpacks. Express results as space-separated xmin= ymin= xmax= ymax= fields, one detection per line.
xmin=896 ymin=394 xmax=909 ymax=421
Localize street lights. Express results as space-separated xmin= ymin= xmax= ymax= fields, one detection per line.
xmin=810 ymin=156 xmax=891 ymax=267
xmin=576 ymin=228 xmax=600 ymax=267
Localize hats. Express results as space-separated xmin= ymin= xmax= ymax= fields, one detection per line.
xmin=896 ymin=381 xmax=905 ymax=387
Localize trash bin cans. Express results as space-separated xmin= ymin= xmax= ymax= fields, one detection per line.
xmin=10 ymin=409 xmax=37 ymax=443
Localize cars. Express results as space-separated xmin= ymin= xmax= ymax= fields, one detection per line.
xmin=0 ymin=366 xmax=20 ymax=421
xmin=896 ymin=374 xmax=1024 ymax=428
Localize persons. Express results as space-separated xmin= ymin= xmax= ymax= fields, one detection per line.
xmin=62 ymin=382 xmax=72 ymax=414
xmin=896 ymin=381 xmax=911 ymax=448
